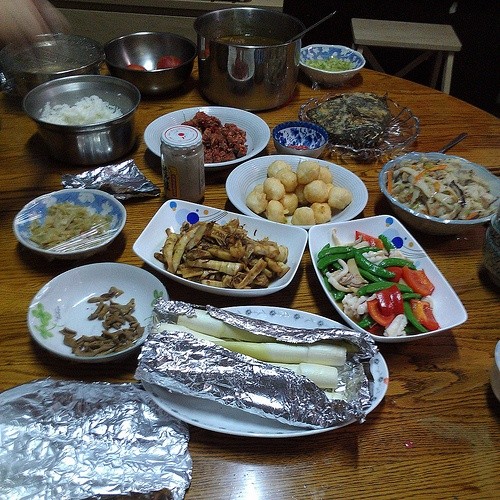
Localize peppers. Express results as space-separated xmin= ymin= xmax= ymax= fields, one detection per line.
xmin=355 ymin=231 xmax=440 ymax=335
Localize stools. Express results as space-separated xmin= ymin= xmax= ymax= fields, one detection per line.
xmin=351 ymin=17 xmax=463 ymax=95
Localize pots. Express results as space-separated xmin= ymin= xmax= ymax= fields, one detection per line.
xmin=193 ymin=6 xmax=307 ymax=111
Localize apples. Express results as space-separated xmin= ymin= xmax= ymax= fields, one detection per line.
xmin=126 ymin=54 xmax=182 ymax=70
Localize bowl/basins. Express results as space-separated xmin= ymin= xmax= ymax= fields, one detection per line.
xmin=22 ymin=73 xmax=141 ymax=166
xmin=272 ymin=121 xmax=329 ymax=159
xmin=226 ymin=154 xmax=368 ymax=229
xmin=377 ymin=152 xmax=500 ymax=236
xmin=143 ymin=105 xmax=271 ymax=170
xmin=103 ymin=30 xmax=198 ymax=98
xmin=0 ymin=32 xmax=105 ymax=100
xmin=13 ymin=187 xmax=127 ymax=260
xmin=298 ymin=92 xmax=419 ymax=162
xmin=300 ymin=44 xmax=366 ymax=85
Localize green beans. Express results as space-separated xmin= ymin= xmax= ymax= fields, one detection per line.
xmin=317 ymin=235 xmax=429 ymax=334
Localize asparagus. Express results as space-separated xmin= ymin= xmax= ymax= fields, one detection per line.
xmin=174 ymin=310 xmax=348 ymax=388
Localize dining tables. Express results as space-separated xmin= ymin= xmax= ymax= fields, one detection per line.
xmin=0 ymin=56 xmax=500 ymax=500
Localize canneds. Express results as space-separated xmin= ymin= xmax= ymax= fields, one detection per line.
xmin=160 ymin=124 xmax=206 ymax=203
xmin=483 ymin=212 xmax=500 ymax=280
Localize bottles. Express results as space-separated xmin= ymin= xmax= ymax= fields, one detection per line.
xmin=482 ymin=211 xmax=500 ymax=280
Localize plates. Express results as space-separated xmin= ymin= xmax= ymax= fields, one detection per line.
xmin=132 ymin=198 xmax=308 ymax=297
xmin=309 ymin=214 xmax=469 ymax=343
xmin=141 ymin=306 xmax=390 ymax=437
xmin=27 ymin=262 xmax=169 ymax=362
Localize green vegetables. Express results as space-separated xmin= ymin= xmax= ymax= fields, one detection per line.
xmin=304 ymin=58 xmax=352 ymax=71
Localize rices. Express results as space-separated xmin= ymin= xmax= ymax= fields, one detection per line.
xmin=40 ymin=94 xmax=125 ymax=125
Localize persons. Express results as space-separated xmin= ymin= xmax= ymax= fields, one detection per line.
xmin=0 ymin=0 xmax=71 ymax=67
